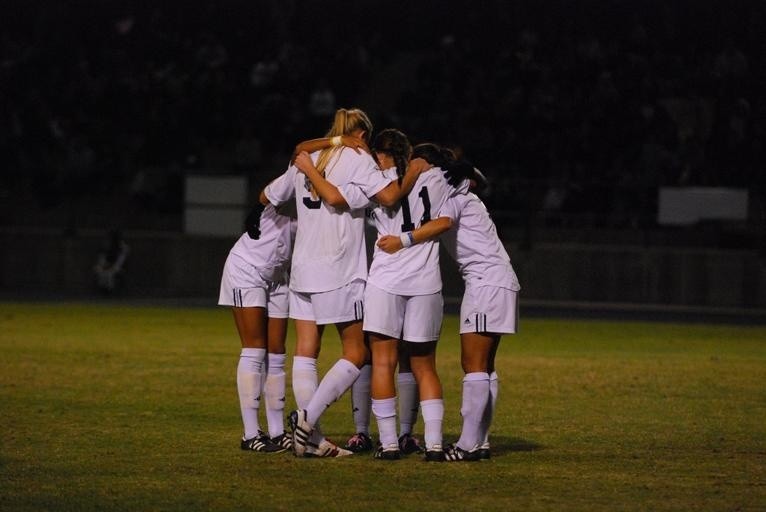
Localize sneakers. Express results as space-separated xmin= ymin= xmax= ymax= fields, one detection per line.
xmin=441 ymin=446 xmax=482 ymax=463
xmin=397 ymin=434 xmax=421 ymax=453
xmin=241 ymin=429 xmax=289 ymax=455
xmin=343 ymin=432 xmax=373 ymax=456
xmin=286 ymin=407 xmax=318 ymax=458
xmin=372 ymin=446 xmax=400 ymax=461
xmin=425 ymin=444 xmax=446 ymax=464
xmin=476 ymin=448 xmax=491 ymax=461
xmin=302 ymin=437 xmax=354 ymax=458
xmin=269 ymin=429 xmax=293 ymax=452
